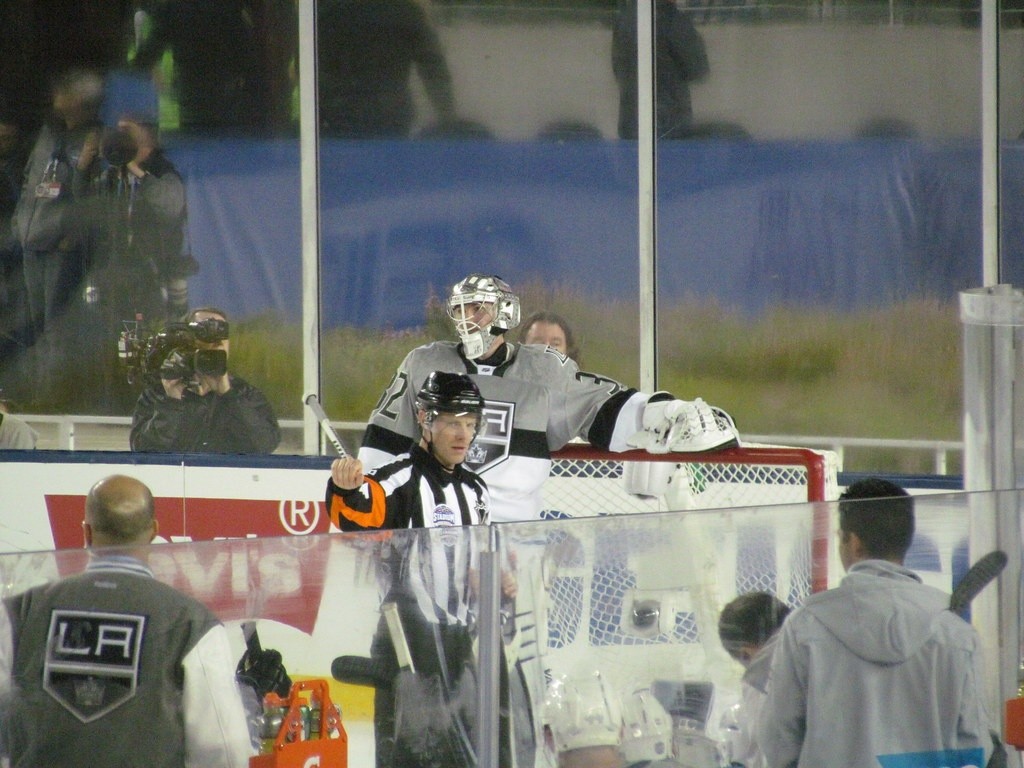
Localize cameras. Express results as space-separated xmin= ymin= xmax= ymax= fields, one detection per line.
xmin=102 ymin=133 xmax=140 ymax=164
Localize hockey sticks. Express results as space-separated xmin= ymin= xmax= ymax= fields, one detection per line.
xmin=945 ymin=547 xmax=1007 ymax=617
xmin=304 ymin=393 xmax=349 ymax=459
xmin=379 ymin=599 xmax=416 ymax=670
xmin=241 ymin=620 xmax=266 ymax=660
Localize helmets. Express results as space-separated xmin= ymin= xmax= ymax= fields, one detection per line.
xmin=416 ymin=370 xmax=485 ymax=413
xmin=446 ymin=274 xmax=522 ymax=359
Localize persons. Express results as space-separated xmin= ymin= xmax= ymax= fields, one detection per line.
xmin=324 ymin=272 xmax=1024 ymax=768
xmin=1 ymin=0 xmax=456 ymax=454
xmin=1 ymin=473 xmax=249 ymax=768
xmin=611 ymin=0 xmax=714 ymax=140
